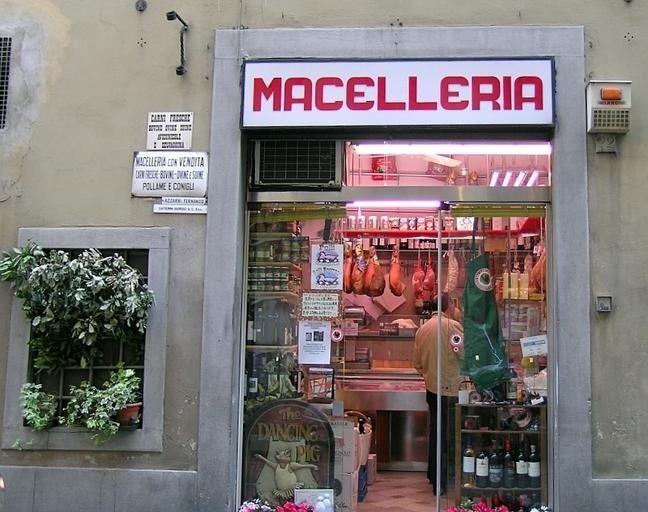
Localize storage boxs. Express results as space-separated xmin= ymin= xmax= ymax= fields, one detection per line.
xmin=326 ymin=416 xmax=359 ymax=512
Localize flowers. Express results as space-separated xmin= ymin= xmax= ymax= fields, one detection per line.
xmin=238 ymin=498 xmax=315 ymax=512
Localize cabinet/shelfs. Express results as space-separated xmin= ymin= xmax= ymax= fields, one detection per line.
xmin=454 ymin=403 xmax=547 ymax=510
xmin=245 ymin=232 xmax=311 ymax=402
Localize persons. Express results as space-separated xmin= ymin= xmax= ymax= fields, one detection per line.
xmin=413 ymin=292 xmax=467 ymax=496
xmin=254 ymin=449 xmax=320 ymax=504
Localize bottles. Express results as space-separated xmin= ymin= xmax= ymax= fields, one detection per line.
xmin=247 ymin=307 xmax=297 ymax=346
xmin=244 ymin=352 xmax=304 ymax=399
xmin=463 ymin=434 xmax=541 ymax=489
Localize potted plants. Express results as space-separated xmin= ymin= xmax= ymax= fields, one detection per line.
xmin=19 ymin=361 xmax=143 ymax=449
